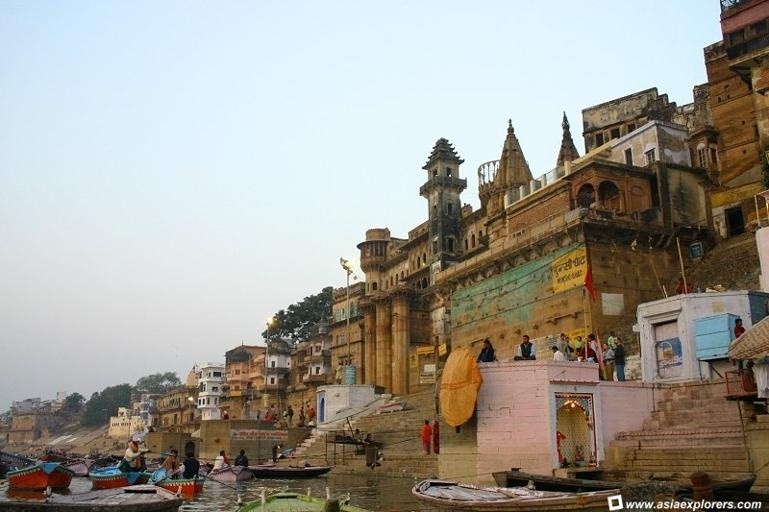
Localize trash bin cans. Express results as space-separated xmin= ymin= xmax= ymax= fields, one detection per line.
xmin=365 ymin=444 xmax=376 ymax=470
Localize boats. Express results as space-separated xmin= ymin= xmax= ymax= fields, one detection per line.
xmin=410 ymin=474 xmax=624 ymax=512
xmin=0 ymin=447 xmax=215 ymax=512
xmin=490 ymin=468 xmax=758 ymax=512
xmin=232 ymin=486 xmax=382 ymax=512
xmin=439 ymin=346 xmax=484 ymax=428
xmin=247 ymin=464 xmax=335 ymax=480
xmin=208 ymin=465 xmax=254 ymax=483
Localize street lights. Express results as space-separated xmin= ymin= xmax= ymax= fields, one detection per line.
xmin=262 ymin=311 xmax=274 ymax=409
xmin=339 ymin=257 xmax=354 ymax=386
xmin=188 ymin=396 xmax=194 ymax=437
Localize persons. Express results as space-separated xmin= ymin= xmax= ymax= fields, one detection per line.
xmin=166 ymin=449 xmax=200 ymax=478
xmin=234 ymin=449 xmax=248 ymax=466
xmin=220 ymin=449 xmax=230 ymax=464
xmin=125 ymin=437 xmax=149 ymax=470
xmin=265 ymin=402 xmax=317 ymax=427
xmin=549 ymin=330 xmax=627 ymax=381
xmin=364 ymin=431 xmax=378 ymax=468
xmin=355 ymin=428 xmax=366 ymax=448
xmin=734 ymin=317 xmax=748 ymax=339
xmin=514 ymin=335 xmax=536 ymax=361
xmin=476 ymin=339 xmax=496 ymax=362
xmin=270 ymin=441 xmax=284 ymax=463
xmin=161 ymin=447 xmax=180 ymax=471
xmin=223 ymin=410 xmax=229 ymax=420
xmin=421 ymin=419 xmax=432 ymax=455
